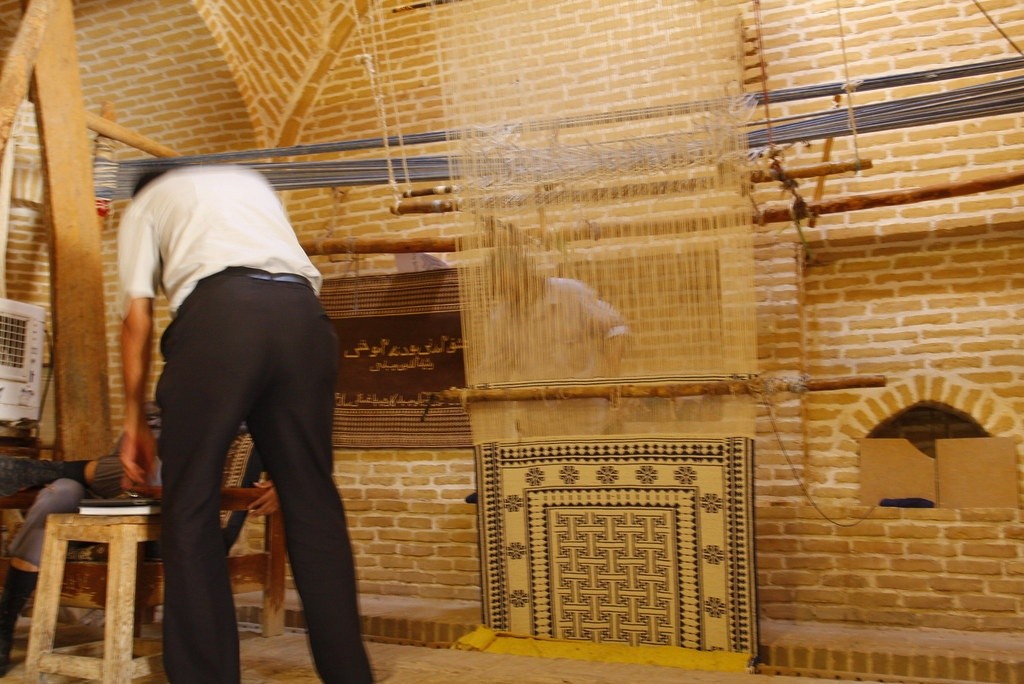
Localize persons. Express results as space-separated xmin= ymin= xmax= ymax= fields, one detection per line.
xmin=112 ymin=159 xmax=376 ymax=684
xmin=486 ymin=266 xmax=636 ymax=381
xmin=0 ymin=416 xmax=283 ymax=675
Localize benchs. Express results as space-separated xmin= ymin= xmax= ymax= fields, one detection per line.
xmin=0 ymin=486 xmax=286 ymax=639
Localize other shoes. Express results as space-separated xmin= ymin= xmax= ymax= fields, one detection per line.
xmin=0 ymin=456 xmax=64 ymax=496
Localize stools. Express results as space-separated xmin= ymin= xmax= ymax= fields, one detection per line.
xmin=24 ymin=513 xmax=165 ymax=684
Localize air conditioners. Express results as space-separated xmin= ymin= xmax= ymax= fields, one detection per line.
xmin=0 ymin=297 xmax=45 ymax=422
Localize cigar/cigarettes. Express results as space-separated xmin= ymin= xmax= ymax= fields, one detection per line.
xmin=248 ymin=509 xmax=257 ymax=514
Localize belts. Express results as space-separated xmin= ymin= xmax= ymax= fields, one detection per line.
xmin=224 ymin=266 xmax=312 ymax=289
